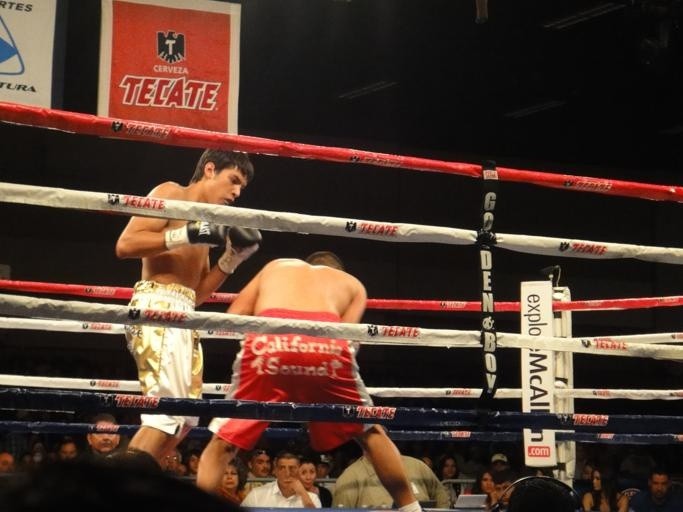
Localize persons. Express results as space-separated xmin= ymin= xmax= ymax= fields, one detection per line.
xmin=192 ymin=250 xmax=423 ymax=511
xmin=113 ymin=146 xmax=262 ymax=471
xmin=1 ymin=412 xmax=682 ymax=511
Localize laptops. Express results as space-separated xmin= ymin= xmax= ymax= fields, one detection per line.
xmin=452 ymin=493 xmax=488 ymax=508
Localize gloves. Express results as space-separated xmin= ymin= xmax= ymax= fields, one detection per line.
xmin=164 ymin=221 xmax=262 ymax=274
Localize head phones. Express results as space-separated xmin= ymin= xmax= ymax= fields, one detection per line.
xmin=491 ymin=475 xmax=586 ymax=512
